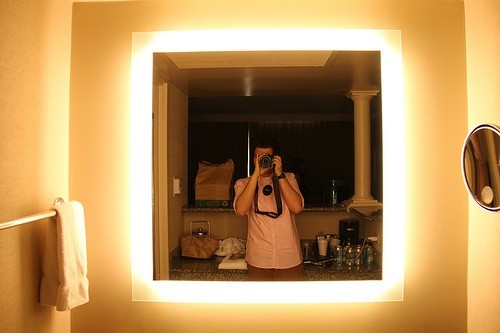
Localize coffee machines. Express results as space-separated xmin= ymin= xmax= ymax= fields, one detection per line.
xmin=340 ymin=218 xmax=359 ymax=245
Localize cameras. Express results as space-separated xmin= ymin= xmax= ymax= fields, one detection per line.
xmin=260 ymin=155 xmax=275 ymax=167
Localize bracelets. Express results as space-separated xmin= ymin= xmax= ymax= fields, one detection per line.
xmin=278 ymin=173 xmax=286 ymax=179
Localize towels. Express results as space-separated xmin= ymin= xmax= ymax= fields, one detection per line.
xmin=40 ymin=200 xmax=89 ymax=312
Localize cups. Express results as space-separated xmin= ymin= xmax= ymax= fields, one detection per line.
xmin=317 ymin=240 xmax=328 ymax=256
xmin=330 ymin=239 xmax=340 ymax=255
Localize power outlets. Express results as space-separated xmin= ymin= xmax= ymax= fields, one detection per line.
xmin=375 ymin=233 xmax=379 ymax=244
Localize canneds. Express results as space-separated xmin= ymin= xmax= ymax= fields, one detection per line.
xmin=335 ymin=245 xmax=375 ymax=266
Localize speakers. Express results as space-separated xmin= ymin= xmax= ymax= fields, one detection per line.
xmin=339 ymin=219 xmax=359 ymax=245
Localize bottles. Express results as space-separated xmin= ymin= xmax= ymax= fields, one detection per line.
xmin=302 ymin=240 xmax=309 ymax=260
xmin=335 ymin=245 xmax=342 ymax=271
xmin=343 ymin=238 xmax=353 ymax=271
xmin=364 ymin=238 xmax=376 ymax=269
xmin=329 ymin=180 xmax=336 ymax=208
xmin=355 ymin=240 xmax=363 ymax=271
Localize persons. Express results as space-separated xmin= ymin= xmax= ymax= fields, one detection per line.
xmin=233 ymin=138 xmax=304 ymax=282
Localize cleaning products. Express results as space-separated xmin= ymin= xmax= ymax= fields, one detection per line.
xmin=363 ymin=242 xmax=379 ymax=270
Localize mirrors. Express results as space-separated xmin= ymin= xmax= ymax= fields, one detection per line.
xmin=132 ymin=29 xmax=405 ymax=302
xmin=462 ymin=123 xmax=500 ymax=212
